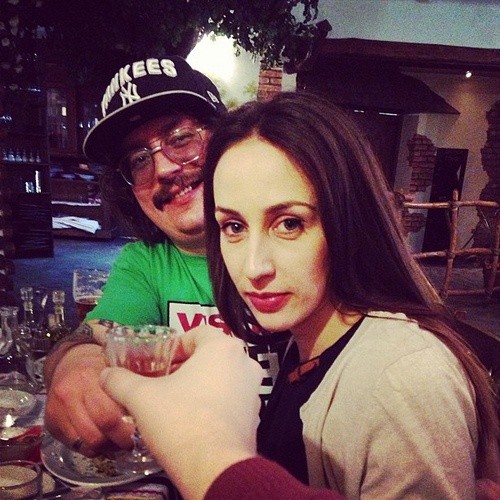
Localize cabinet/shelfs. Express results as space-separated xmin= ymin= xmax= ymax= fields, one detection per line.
xmin=0 ymin=72 xmax=141 ymax=289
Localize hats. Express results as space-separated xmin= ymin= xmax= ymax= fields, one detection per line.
xmin=82 ymin=56 xmax=227 ymax=160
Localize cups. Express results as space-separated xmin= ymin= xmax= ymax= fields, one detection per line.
xmin=72 ymin=268 xmax=112 ymax=326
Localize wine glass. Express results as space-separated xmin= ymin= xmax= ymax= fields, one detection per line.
xmin=0 ymin=379 xmax=38 ymax=439
xmin=104 ymin=324 xmax=178 ymax=474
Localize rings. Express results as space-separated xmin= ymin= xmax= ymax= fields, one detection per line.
xmin=72 ymin=436 xmax=85 ymax=450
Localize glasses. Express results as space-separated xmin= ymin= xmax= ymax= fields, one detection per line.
xmin=115 ymin=125 xmax=209 ymax=187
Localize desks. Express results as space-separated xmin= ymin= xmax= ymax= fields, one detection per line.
xmin=0 ymin=367 xmax=171 ymax=500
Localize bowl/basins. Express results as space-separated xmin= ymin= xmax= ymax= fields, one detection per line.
xmin=0 ymin=460 xmax=41 ymax=500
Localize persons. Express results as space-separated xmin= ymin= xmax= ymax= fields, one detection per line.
xmin=42 ymin=53 xmax=287 ymax=458
xmin=202 ymin=91 xmax=500 ymax=500
xmin=99 ymin=325 xmax=345 ymax=500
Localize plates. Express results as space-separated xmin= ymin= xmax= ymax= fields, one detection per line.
xmin=23 ymin=394 xmax=47 ymax=426
xmin=40 ymin=416 xmax=162 ymax=487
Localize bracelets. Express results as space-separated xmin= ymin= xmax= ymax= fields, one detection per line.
xmin=42 ymin=334 xmax=103 ymax=389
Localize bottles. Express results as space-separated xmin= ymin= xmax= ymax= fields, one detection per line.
xmin=25 ymin=182 xmax=34 ymax=193
xmin=2 ymin=145 xmax=41 ymax=163
xmin=0 ymin=305 xmax=40 ymax=394
xmin=47 ymin=289 xmax=92 ymax=346
xmin=12 ymin=287 xmax=49 ymax=355
